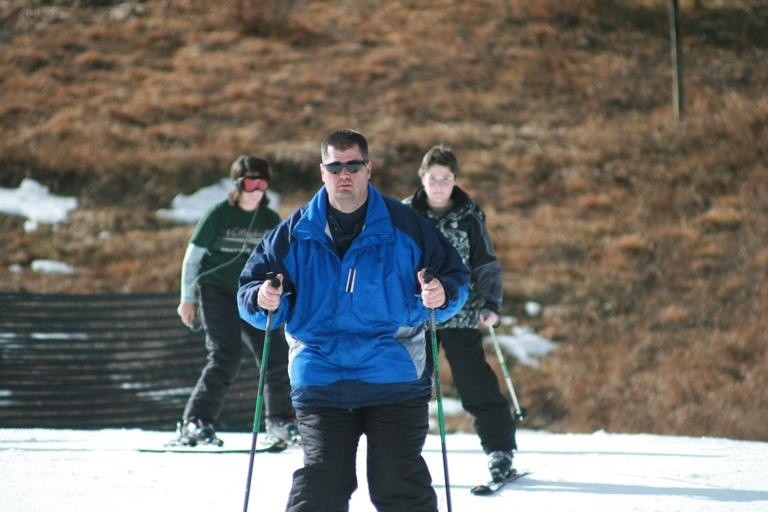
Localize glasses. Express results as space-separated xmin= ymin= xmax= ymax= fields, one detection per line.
xmin=234 ymin=176 xmax=270 ymax=191
xmin=322 ymin=160 xmax=367 ymax=174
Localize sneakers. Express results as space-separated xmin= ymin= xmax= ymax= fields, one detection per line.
xmin=490 ymin=451 xmax=514 ymax=476
xmin=266 ymin=419 xmax=302 ymax=445
xmin=181 ymin=419 xmax=214 ymax=442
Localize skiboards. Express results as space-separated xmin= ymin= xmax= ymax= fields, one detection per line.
xmin=470 ymin=468 xmax=531 ymax=494
xmin=69 ymin=418 xmax=302 ymax=455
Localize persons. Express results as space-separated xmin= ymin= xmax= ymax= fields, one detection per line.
xmin=396 ymin=147 xmax=517 ymax=485
xmin=234 ymin=131 xmax=472 ymax=511
xmin=176 ymin=155 xmax=306 ymax=445
